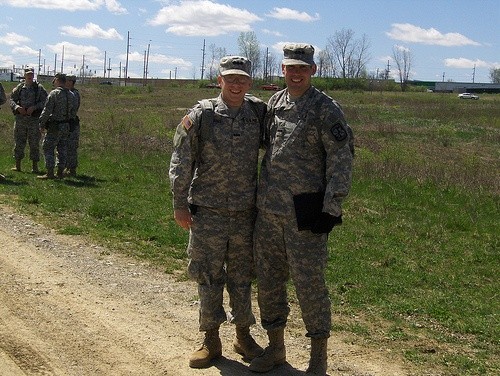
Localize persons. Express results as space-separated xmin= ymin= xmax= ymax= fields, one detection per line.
xmin=249 ymin=42 xmax=355 ymax=376
xmin=0 ymin=83 xmax=7 ymax=105
xmin=36 ymin=73 xmax=74 ymax=179
xmin=65 ymin=75 xmax=81 ymax=178
xmin=168 ymin=55 xmax=269 ymax=367
xmin=10 ymin=69 xmax=49 ymax=173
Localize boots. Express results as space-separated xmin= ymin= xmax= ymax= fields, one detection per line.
xmin=31 ymin=160 xmax=39 ymax=173
xmin=302 ymin=337 xmax=328 ymax=376
xmin=11 ymin=159 xmax=21 ymax=171
xmin=189 ymin=329 xmax=222 ymax=369
xmin=232 ymin=326 xmax=264 ymax=360
xmin=248 ymin=327 xmax=287 ymax=373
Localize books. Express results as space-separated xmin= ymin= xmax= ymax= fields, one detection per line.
xmin=292 ymin=192 xmax=342 ymax=231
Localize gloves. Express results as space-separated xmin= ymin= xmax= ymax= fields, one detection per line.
xmin=311 ymin=216 xmax=333 ymax=234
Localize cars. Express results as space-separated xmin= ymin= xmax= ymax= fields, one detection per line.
xmin=256 ymin=83 xmax=281 ymax=91
xmin=99 ymin=81 xmax=114 ymax=86
xmin=197 ymin=83 xmax=222 ymax=89
xmin=457 ymin=92 xmax=480 ymax=100
xmin=427 ymin=89 xmax=433 ymax=93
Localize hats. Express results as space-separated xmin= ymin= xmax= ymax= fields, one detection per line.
xmin=218 ymin=55 xmax=253 ymax=78
xmin=24 ymin=66 xmax=35 ymax=75
xmin=65 ymin=74 xmax=77 ymax=81
xmin=281 ymin=44 xmax=315 ymax=66
xmin=51 ymin=73 xmax=66 ymax=84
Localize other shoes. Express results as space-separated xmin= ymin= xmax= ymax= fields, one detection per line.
xmin=53 ymin=172 xmax=63 ymax=179
xmin=36 ymin=171 xmax=54 ymax=179
xmin=63 ymin=167 xmax=77 ymax=176
